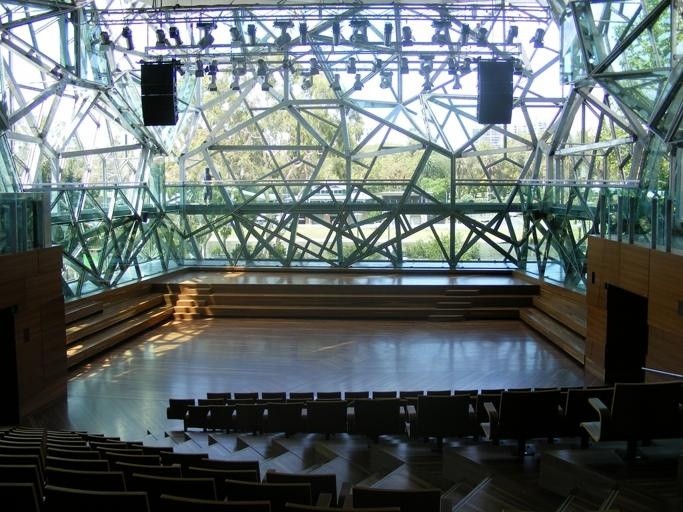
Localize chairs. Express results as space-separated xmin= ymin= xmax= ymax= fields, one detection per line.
xmin=164 ymin=382 xmax=682 ymax=460
xmin=1 ymin=423 xmax=452 ymax=510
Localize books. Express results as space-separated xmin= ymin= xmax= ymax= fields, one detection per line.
xmin=1 ymin=190 xmax=54 ymax=252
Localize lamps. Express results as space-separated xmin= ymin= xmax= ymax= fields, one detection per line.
xmin=331 ymin=14 xmax=416 ymax=46
xmin=329 ymin=54 xmax=366 ymax=93
xmin=149 ymin=16 xmax=183 ymax=48
xmin=225 ymin=52 xmax=249 ymax=92
xmin=269 ymin=11 xmax=309 ymax=51
xmin=144 ymin=51 xmax=186 ymax=78
xmin=96 ymin=14 xmax=137 ymax=53
xmin=429 ymin=16 xmax=548 ymax=49
xmin=281 ymin=56 xmax=320 ymax=91
xmin=194 ymin=54 xmax=220 ymax=92
xmin=193 ymin=13 xmax=218 ymax=51
xmin=229 ymin=13 xmax=258 ymax=49
xmin=257 ymin=56 xmax=274 ymax=94
xmin=371 ymin=54 xmax=411 ymax=89
xmin=416 ymin=53 xmax=526 ymax=93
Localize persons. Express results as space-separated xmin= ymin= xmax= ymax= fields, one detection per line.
xmin=203 ymin=167 xmax=213 ymax=203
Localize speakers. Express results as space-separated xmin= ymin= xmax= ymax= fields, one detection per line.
xmin=477 ymin=61 xmax=513 ymax=124
xmin=141 ymin=62 xmax=178 ymax=126
